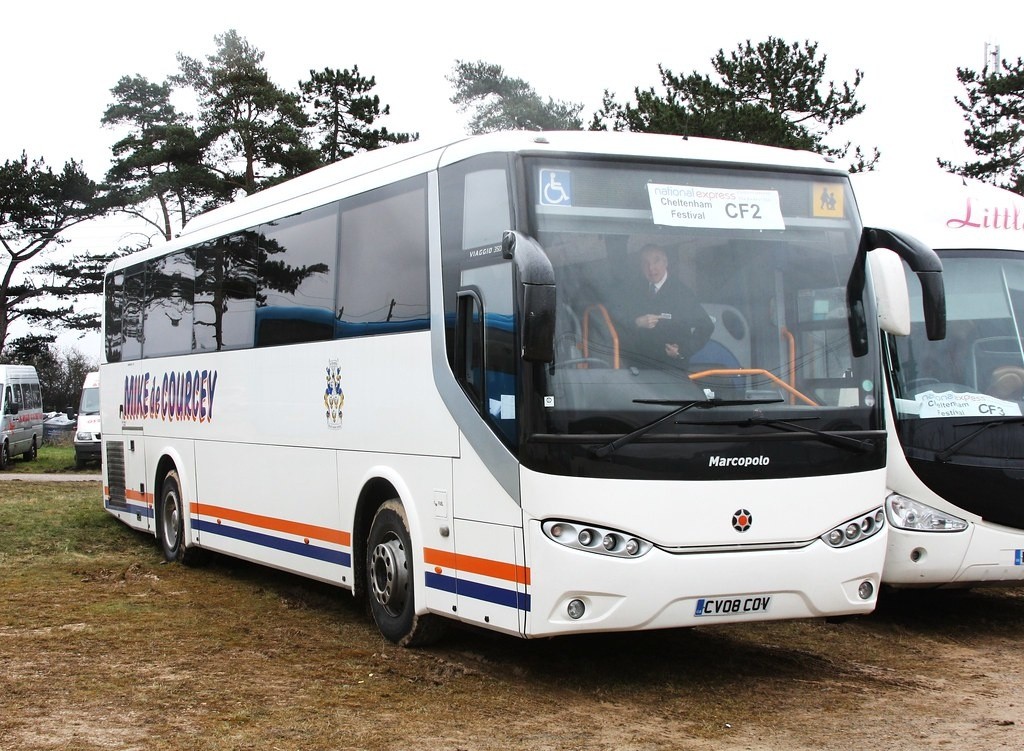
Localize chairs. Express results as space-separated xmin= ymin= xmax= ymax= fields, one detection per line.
xmin=697 ymin=302 xmax=754 ymax=395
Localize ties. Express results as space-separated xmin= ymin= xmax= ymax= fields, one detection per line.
xmin=649 ymin=284 xmax=656 ymax=294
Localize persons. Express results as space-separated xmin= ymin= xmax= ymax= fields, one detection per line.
xmin=606 ymin=242 xmax=717 ymax=374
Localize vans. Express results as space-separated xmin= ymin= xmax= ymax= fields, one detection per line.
xmin=0 ymin=364 xmax=44 ymax=470
xmin=73 ymin=371 xmax=101 ymax=466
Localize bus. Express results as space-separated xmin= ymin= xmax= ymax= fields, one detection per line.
xmin=100 ymin=129 xmax=946 ymax=649
xmin=849 ymin=167 xmax=1023 ymax=584
